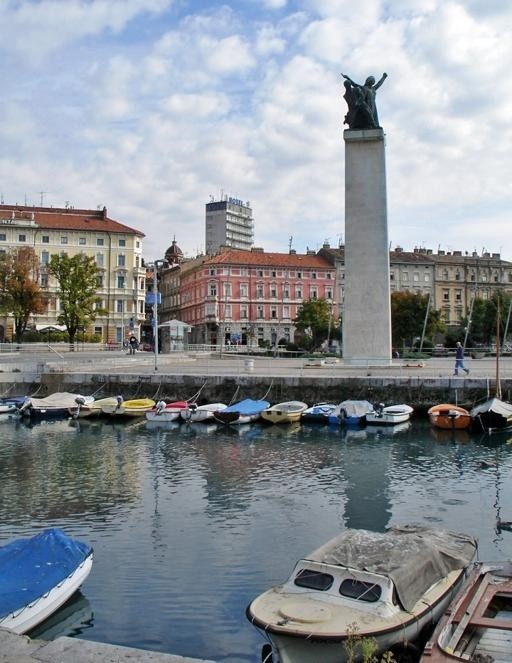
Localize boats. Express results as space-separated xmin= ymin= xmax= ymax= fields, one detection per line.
xmin=180 ymin=402 xmax=228 ymax=424
xmin=0 ymin=395 xmax=27 ymax=413
xmin=260 ymin=400 xmax=309 ymax=425
xmin=418 ymin=563 xmax=512 ymax=663
xmin=144 ymin=399 xmax=190 ymax=422
xmin=427 ymin=403 xmax=471 ymax=428
xmin=0 ymin=531 xmax=96 ymax=638
xmin=100 ymin=395 xmax=156 ymax=417
xmin=68 ymin=395 xmax=124 ymax=419
xmin=366 ymin=402 xmax=414 ymax=424
xmin=16 ymin=391 xmax=95 ymax=418
xmin=212 ymin=398 xmax=271 ymax=425
xmin=301 ymin=401 xmax=337 ymax=423
xmin=28 ymin=591 xmax=93 ymax=640
xmin=246 ymin=525 xmax=483 ymax=663
xmin=328 ymin=400 xmax=374 ymax=426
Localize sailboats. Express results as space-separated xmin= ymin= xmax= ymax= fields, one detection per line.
xmin=471 ymin=296 xmax=512 ymax=430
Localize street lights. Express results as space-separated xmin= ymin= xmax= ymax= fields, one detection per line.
xmin=150 ymin=257 xmax=178 ymax=370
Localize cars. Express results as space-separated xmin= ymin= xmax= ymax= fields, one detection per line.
xmin=433 ymin=343 xmax=448 ymax=355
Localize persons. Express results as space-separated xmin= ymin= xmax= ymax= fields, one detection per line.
xmin=452 ymin=339 xmax=471 ymax=375
xmin=129 ymin=331 xmax=139 ymax=352
xmin=341 ymin=73 xmax=388 ymax=129
